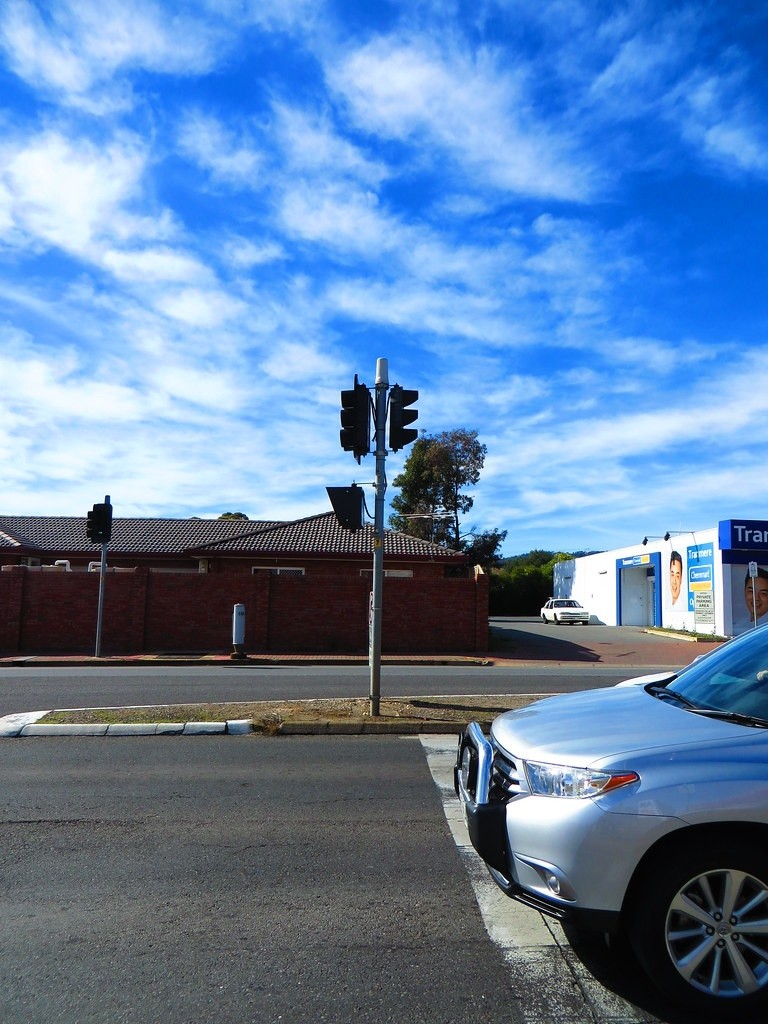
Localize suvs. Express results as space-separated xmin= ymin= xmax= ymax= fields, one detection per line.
xmin=451 ymin=618 xmax=768 ymax=1024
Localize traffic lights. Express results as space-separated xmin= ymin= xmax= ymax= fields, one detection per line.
xmin=388 ymin=385 xmax=420 ymax=451
xmin=86 ymin=504 xmax=113 ymax=544
xmin=325 ymin=486 xmax=366 ymax=531
xmin=339 ymin=374 xmax=372 ymax=466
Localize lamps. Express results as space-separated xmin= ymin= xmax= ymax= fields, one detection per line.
xmin=665 ymin=531 xmax=693 ymax=541
xmin=642 ymin=536 xmax=664 ymax=545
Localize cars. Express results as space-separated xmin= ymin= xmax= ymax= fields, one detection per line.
xmin=540 ymin=597 xmax=590 ymax=626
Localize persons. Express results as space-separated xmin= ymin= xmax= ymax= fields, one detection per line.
xmin=733 ymin=567 xmax=768 ymax=634
xmin=669 ymin=551 xmax=682 ymax=606
